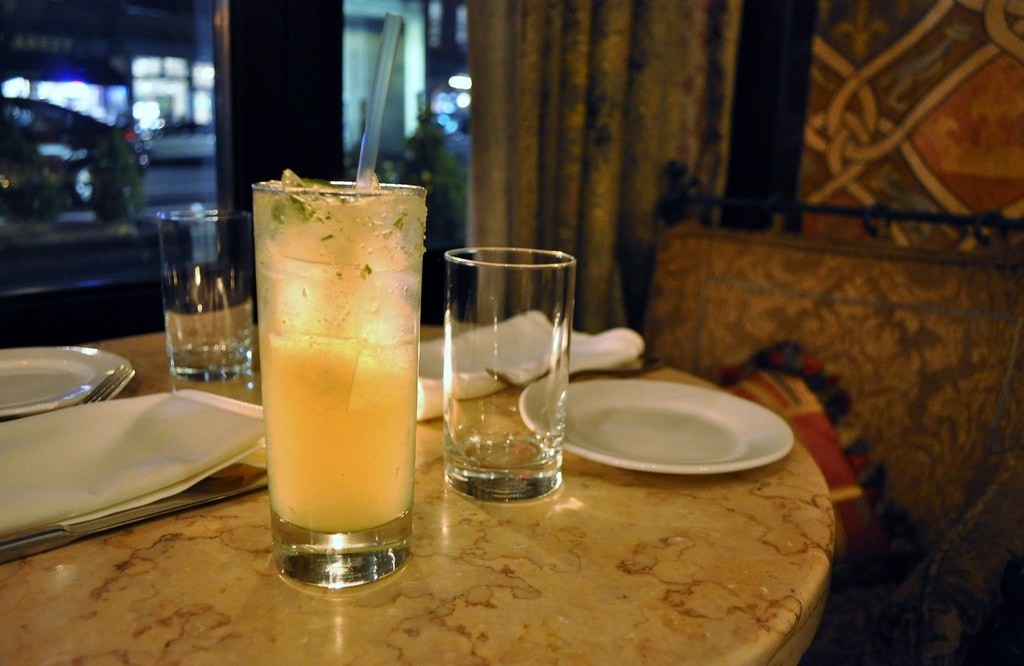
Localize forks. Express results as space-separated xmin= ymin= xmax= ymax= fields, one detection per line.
xmin=484 ymin=353 xmax=670 ymax=390
xmin=75 ymin=364 xmax=135 ymax=407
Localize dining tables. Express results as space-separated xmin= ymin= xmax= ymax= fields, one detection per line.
xmin=2 ymin=321 xmax=838 ymax=665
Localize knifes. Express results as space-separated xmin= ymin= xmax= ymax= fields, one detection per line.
xmin=0 ymin=471 xmax=267 ymax=561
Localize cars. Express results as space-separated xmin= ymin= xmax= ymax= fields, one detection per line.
xmin=0 ymin=96 xmax=144 ymax=225
xmin=138 ymin=122 xmax=215 ymax=166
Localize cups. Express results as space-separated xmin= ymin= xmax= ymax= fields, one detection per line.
xmin=252 ymin=179 xmax=427 ymax=588
xmin=443 ymin=245 xmax=576 ymax=503
xmin=158 ymin=204 xmax=254 ymax=379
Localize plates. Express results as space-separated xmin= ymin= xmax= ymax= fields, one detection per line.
xmin=519 ymin=374 xmax=795 ymax=476
xmin=0 ymin=345 xmax=131 ymax=419
xmin=0 ymin=387 xmax=266 ymax=540
